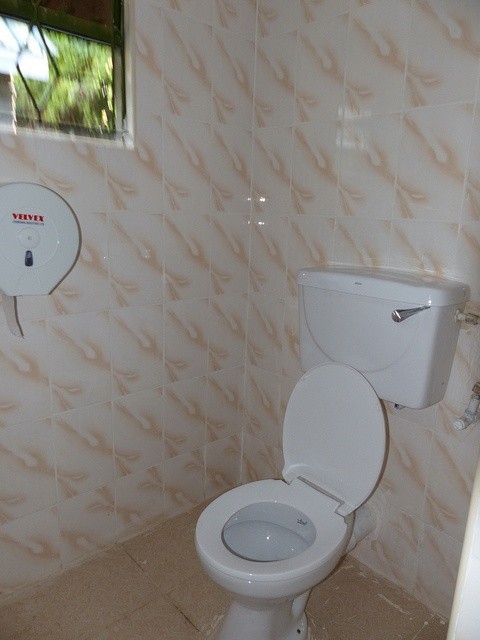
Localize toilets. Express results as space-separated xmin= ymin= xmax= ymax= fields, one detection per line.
xmin=195 ymin=268 xmax=470 ymax=640
